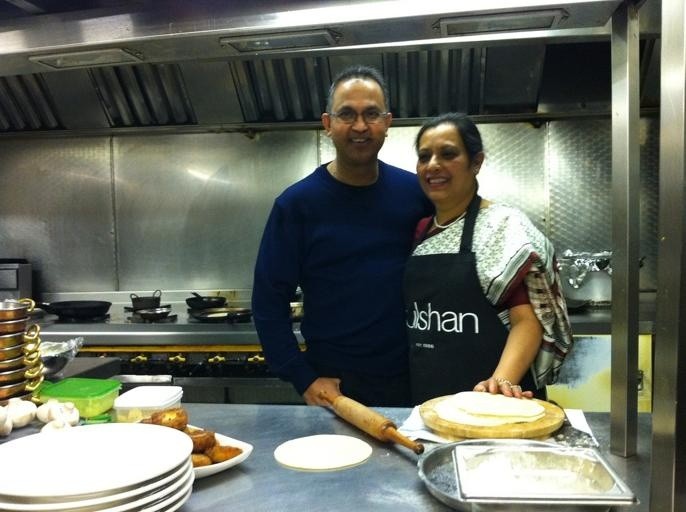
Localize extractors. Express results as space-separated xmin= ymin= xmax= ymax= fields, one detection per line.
xmin=0 ymin=1 xmax=663 ymax=140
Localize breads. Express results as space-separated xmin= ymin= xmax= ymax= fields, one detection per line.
xmin=139 ymin=408 xmax=242 ymax=470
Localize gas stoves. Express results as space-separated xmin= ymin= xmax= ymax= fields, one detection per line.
xmin=29 ymin=309 xmax=308 ymax=379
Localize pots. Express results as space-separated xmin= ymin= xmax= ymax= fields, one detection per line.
xmin=0 ymin=298 xmax=44 ymax=406
xmin=35 ymin=289 xmax=305 ymax=320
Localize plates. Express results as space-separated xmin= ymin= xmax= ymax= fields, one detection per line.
xmin=188 ymin=425 xmax=256 ymax=479
xmin=0 ymin=422 xmax=197 ymax=512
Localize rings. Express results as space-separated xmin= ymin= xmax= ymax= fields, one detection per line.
xmin=510 ymin=384 xmax=522 ymax=392
xmin=499 ymin=379 xmax=512 ymax=388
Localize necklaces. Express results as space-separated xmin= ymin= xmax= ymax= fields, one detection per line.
xmin=433 ymin=210 xmax=467 ymax=229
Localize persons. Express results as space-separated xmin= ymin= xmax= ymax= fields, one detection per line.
xmin=401 ymin=111 xmax=573 ymax=407
xmin=252 ymin=67 xmax=437 ymax=405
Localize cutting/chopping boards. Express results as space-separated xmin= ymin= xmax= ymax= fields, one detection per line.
xmin=417 ymin=391 xmax=566 ymax=442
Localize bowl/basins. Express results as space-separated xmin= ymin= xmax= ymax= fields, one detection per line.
xmin=40 ymin=342 xmax=75 ymax=375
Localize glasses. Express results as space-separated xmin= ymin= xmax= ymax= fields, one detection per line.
xmin=327 ymin=109 xmax=388 ymax=125
